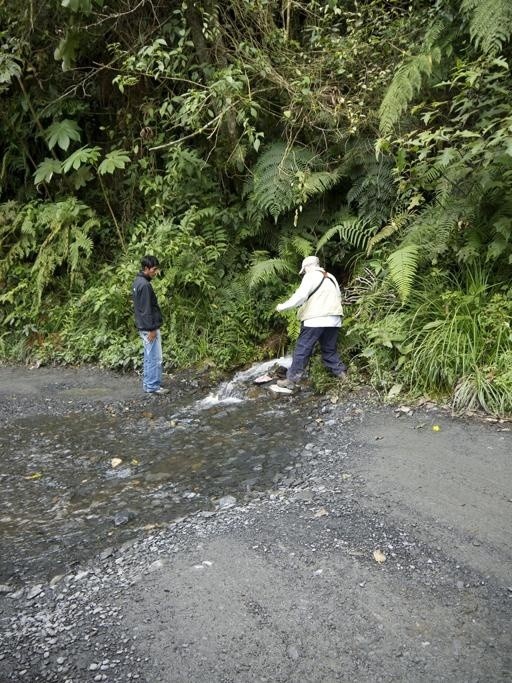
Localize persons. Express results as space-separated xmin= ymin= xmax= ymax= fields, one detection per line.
xmin=133 ymin=253 xmax=172 ymax=396
xmin=274 ymin=253 xmax=353 ymax=392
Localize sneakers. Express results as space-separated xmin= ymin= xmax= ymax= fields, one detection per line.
xmin=154 ymin=387 xmax=169 ymax=395
xmin=277 ymin=379 xmax=296 ymax=389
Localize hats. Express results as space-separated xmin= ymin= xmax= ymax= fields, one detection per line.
xmin=299 ymin=256 xmax=320 ymax=274
